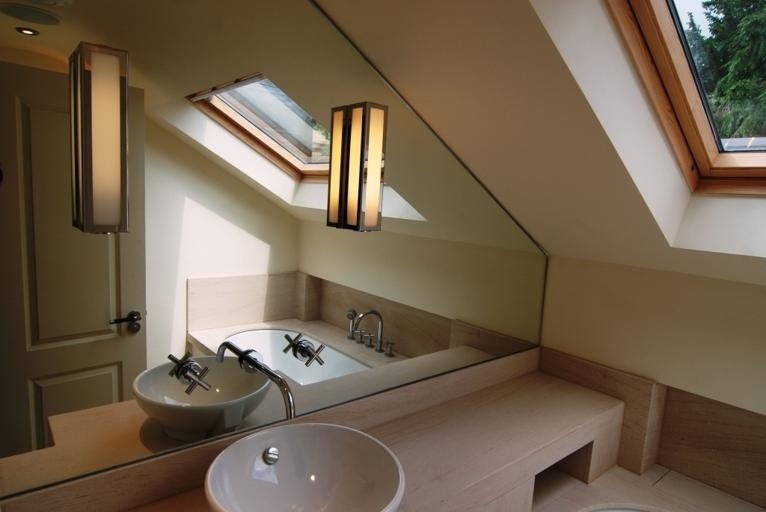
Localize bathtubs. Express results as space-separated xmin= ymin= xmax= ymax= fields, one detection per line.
xmin=223 ymin=328 xmax=372 ymax=384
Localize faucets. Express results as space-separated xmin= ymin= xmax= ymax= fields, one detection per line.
xmin=215 ymin=340 xmax=262 ymax=376
xmin=351 ymin=309 xmax=384 ymax=351
xmin=131 ymin=354 xmax=273 ymax=442
xmin=243 ymin=352 xmax=296 ymax=419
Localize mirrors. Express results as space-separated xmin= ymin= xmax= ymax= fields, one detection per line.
xmin=0 ymin=0 xmax=549 ymax=498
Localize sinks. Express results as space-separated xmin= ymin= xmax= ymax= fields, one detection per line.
xmin=204 ymin=423 xmax=407 ymax=511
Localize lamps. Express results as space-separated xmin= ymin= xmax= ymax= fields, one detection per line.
xmin=342 ymin=102 xmax=389 ymax=233
xmin=68 ymin=50 xmax=75 ymax=228
xmin=74 ymin=41 xmax=129 ymax=234
xmin=327 ymin=105 xmax=347 ymax=228
